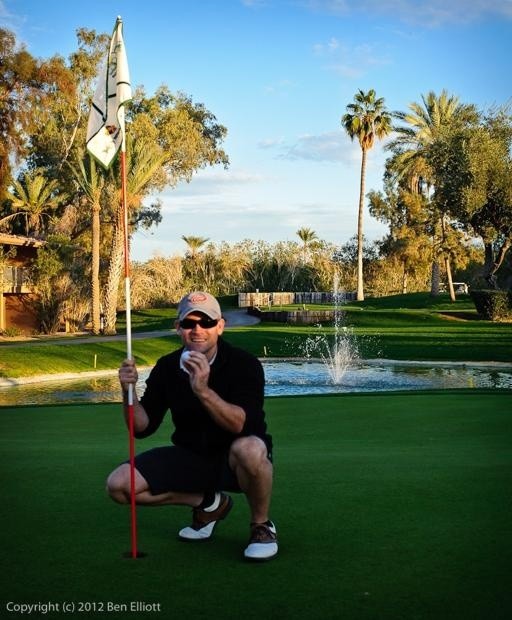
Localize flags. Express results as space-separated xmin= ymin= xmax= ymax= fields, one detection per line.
xmin=86 ymin=21 xmax=133 ymax=171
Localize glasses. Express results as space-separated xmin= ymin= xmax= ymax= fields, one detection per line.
xmin=179 ymin=318 xmax=218 ymax=330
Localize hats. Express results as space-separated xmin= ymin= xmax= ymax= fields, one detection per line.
xmin=178 ymin=291 xmax=222 ymax=321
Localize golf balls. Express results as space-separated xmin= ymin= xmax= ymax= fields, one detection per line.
xmin=180 ymin=350 xmax=190 ymax=362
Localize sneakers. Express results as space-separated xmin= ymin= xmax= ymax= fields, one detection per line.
xmin=178 ymin=491 xmax=234 ymax=543
xmin=242 ymin=520 xmax=280 ymax=562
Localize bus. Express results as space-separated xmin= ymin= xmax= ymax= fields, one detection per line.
xmin=429 ymin=282 xmax=470 ymax=294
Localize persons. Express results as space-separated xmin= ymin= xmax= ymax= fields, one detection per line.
xmin=105 ymin=290 xmax=278 ymax=561
